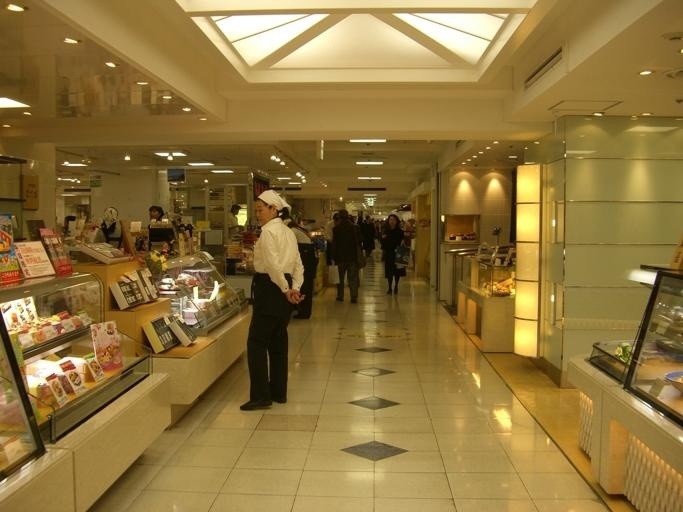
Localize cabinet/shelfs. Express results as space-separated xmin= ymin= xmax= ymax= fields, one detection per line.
xmin=0 ymin=271 xmax=153 ymax=445
xmin=623 ymin=259 xmax=683 ymax=424
xmin=72 ymin=251 xmax=253 ymax=428
xmin=0 ymin=297 xmax=46 ymax=483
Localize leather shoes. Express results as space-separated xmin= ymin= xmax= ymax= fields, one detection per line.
xmin=293 ymin=313 xmax=311 ymax=319
xmin=240 ymin=399 xmax=273 ymax=410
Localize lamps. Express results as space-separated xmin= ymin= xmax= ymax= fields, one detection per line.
xmin=167 ymin=152 xmax=174 ymax=161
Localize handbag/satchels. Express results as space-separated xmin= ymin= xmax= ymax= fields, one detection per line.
xmin=395 ymin=247 xmax=411 ymax=266
xmin=354 ymin=247 xmax=367 ymax=268
xmin=323 ymin=264 xmax=339 ymax=288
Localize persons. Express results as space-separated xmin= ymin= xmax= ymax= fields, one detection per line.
xmin=281 ymin=215 xmax=315 ymax=319
xmin=149 ymin=205 xmax=165 ymax=221
xmin=228 ymin=205 xmax=241 ymax=227
xmin=240 ymin=190 xmax=305 ymax=410
xmin=94 ymin=208 xmax=124 ymax=249
xmin=332 ymin=209 xmax=407 ymax=303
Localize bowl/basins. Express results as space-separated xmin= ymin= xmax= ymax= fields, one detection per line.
xmin=664 ymin=371 xmax=683 ymax=393
